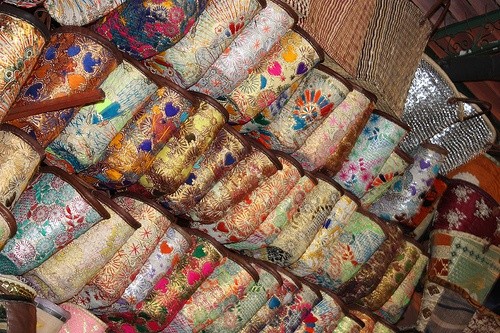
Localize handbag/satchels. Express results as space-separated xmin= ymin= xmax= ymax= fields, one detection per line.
xmin=0 ymin=0 xmax=500 ymax=333
